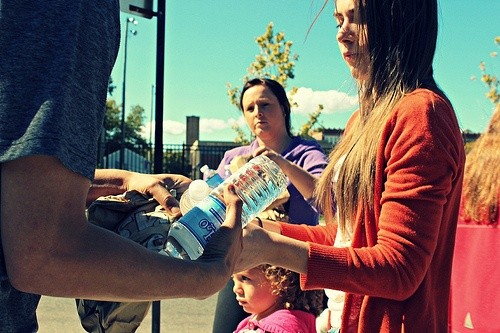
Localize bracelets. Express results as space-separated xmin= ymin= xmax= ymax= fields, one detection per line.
xmin=256 ymin=216 xmax=263 ymax=229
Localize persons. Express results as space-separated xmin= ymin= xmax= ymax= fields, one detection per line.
xmin=233 ymin=263 xmax=327 ymax=333
xmin=233 ymin=0 xmax=465 ymax=333
xmin=1 ymin=0 xmax=242 ymax=333
xmin=212 ymin=78 xmax=327 ymax=333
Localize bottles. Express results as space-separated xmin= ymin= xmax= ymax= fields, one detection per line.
xmin=200 ymin=164 xmax=222 ymax=189
xmin=223 ymin=165 xmax=232 ymax=179
xmin=158 ymin=154 xmax=289 ymax=261
xmin=179 ymin=180 xmax=211 ymax=217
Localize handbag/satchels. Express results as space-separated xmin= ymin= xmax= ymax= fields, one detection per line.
xmin=76 ymin=191 xmax=180 ymax=332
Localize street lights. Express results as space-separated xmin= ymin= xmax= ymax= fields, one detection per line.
xmin=119 ymin=17 xmax=138 ymax=169
